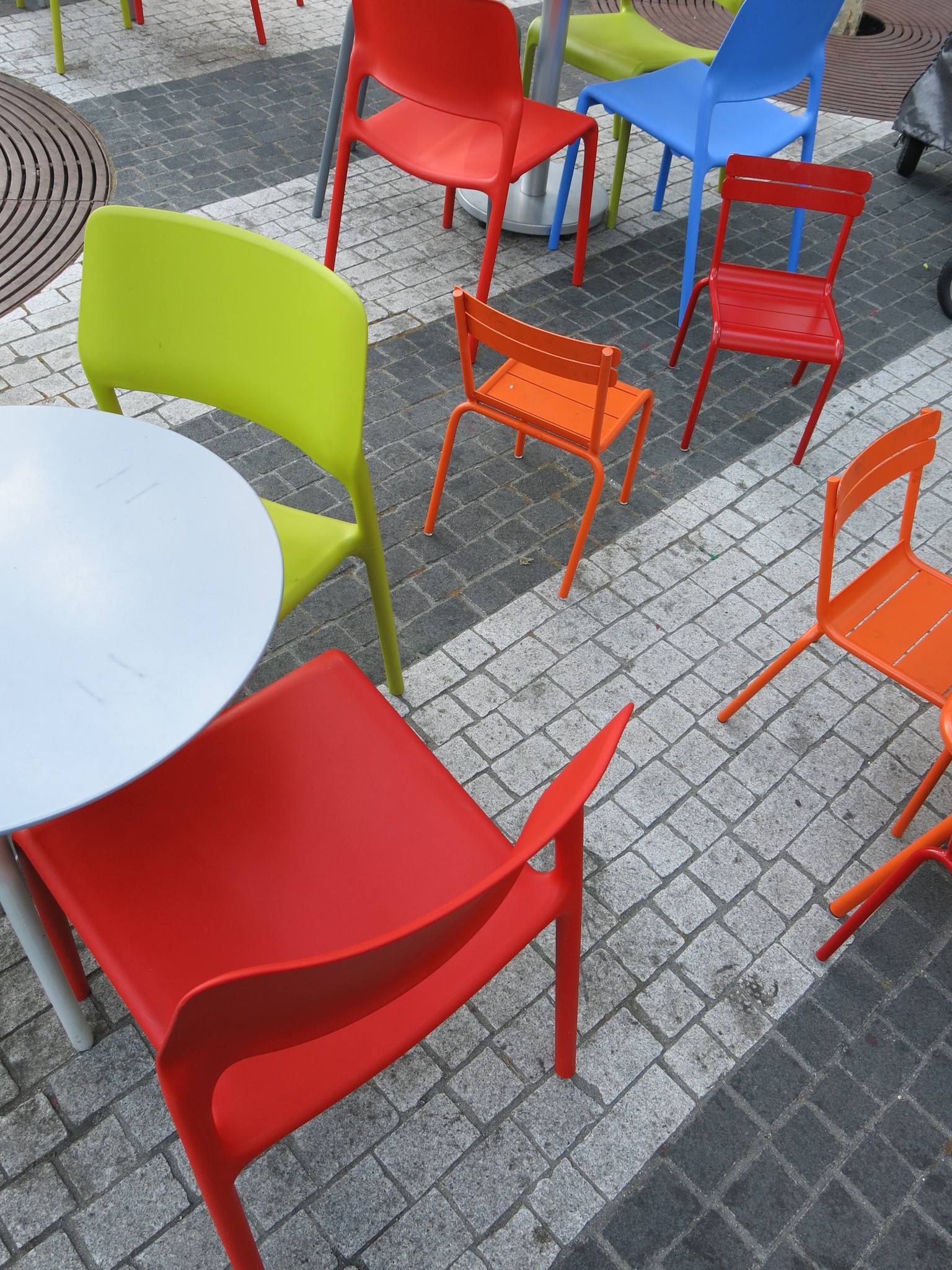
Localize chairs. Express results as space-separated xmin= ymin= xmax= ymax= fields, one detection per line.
xmin=10 ymin=0 xmax=952 ymax=1270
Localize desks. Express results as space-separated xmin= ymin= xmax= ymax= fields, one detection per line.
xmin=0 ymin=404 xmax=284 ymax=1053
